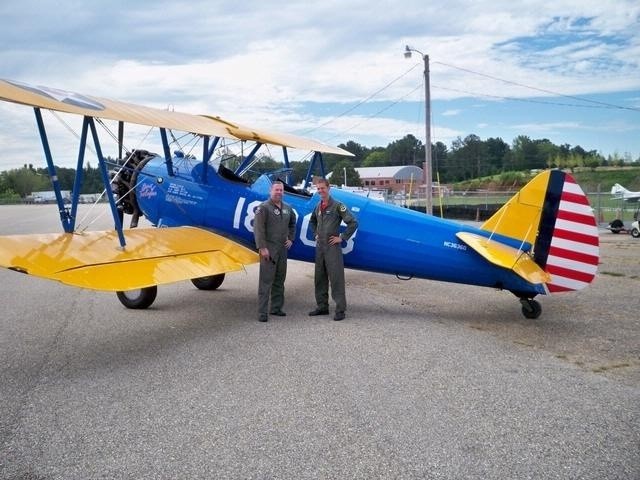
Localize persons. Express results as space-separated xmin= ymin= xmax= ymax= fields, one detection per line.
xmin=251 ymin=181 xmax=297 ymax=322
xmin=308 ymin=177 xmax=359 ymax=321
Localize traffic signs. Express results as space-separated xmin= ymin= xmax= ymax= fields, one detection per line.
xmin=404 ymin=45 xmax=434 ymax=216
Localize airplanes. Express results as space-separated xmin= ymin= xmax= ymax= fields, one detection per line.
xmin=0 ymin=79 xmax=600 ymax=321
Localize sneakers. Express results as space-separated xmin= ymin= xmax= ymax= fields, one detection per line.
xmin=259 ymin=315 xmax=267 ymax=322
xmin=334 ymin=312 xmax=344 ymax=321
xmin=310 ymin=311 xmax=328 ymax=315
xmin=272 ymin=312 xmax=286 ymax=316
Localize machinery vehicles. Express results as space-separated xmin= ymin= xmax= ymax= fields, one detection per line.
xmin=605 ymin=183 xmax=640 ymax=238
